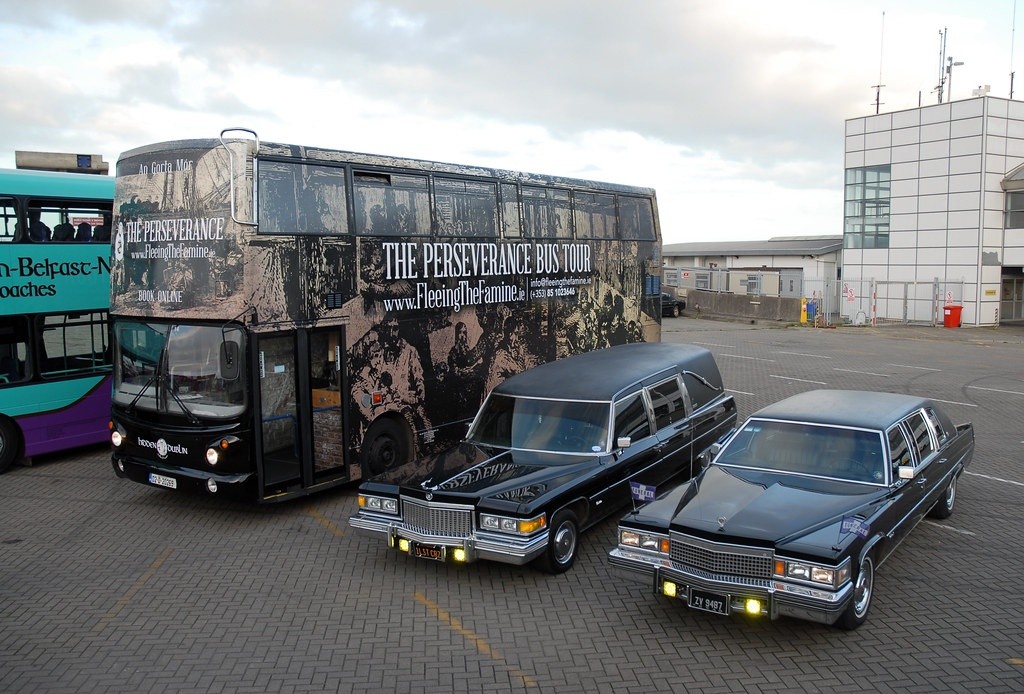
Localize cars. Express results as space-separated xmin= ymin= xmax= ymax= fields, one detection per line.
xmin=345 ymin=341 xmax=739 ymax=575
xmin=607 ymin=388 xmax=976 ymax=631
xmin=662 ymin=292 xmax=686 ymax=318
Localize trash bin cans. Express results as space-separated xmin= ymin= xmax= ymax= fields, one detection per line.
xmin=943 ymin=305 xmax=962 ymax=327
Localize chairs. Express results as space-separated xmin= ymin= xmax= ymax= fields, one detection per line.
xmin=770 ymin=430 xmax=820 ymax=466
xmin=153 ymin=334 xmax=189 ymax=382
xmin=580 ymin=422 xmax=608 ymax=443
xmin=13 ymin=220 xmax=111 ymax=243
xmin=830 ymin=433 xmax=879 ymax=475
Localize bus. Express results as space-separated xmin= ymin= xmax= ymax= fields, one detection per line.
xmin=108 ymin=127 xmax=664 ymax=516
xmin=0 ymin=151 xmax=164 ymax=475
